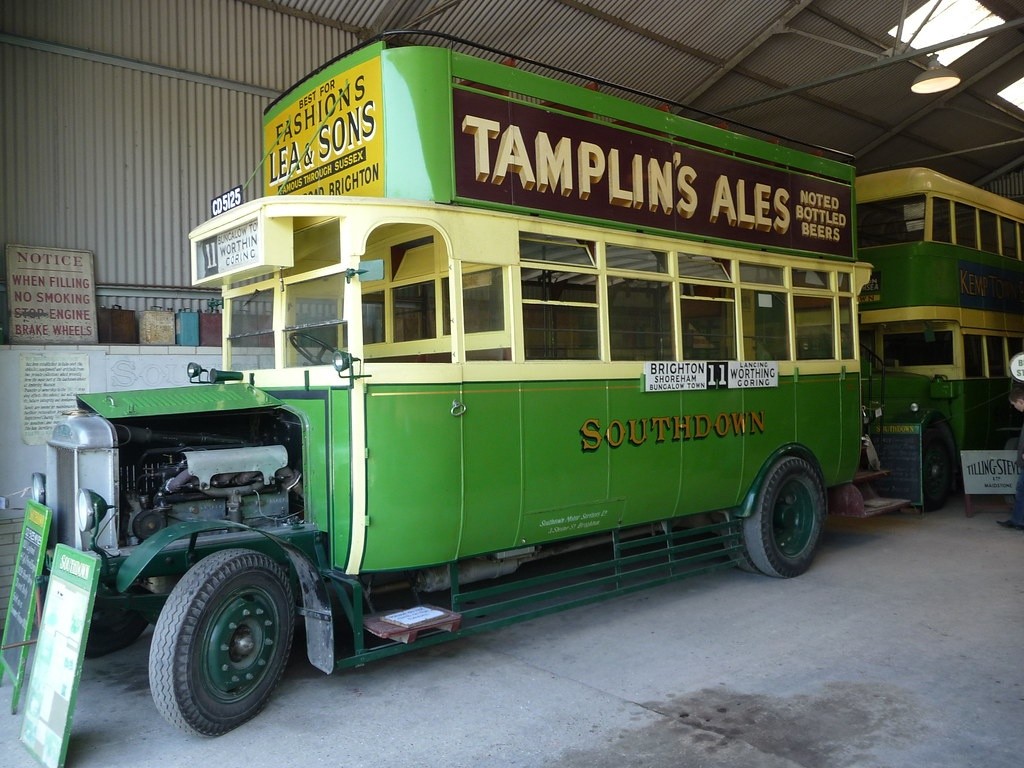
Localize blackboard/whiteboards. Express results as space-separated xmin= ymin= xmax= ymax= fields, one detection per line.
xmin=4 ymin=242 xmax=99 ymax=344
xmin=0 ymin=498 xmax=53 ymax=685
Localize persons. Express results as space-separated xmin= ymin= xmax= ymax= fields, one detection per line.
xmin=997 ymin=388 xmax=1024 ymax=530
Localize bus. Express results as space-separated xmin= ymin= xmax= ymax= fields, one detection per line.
xmin=855 ymin=165 xmax=1024 ymax=513
xmin=31 ymin=27 xmax=913 ymax=745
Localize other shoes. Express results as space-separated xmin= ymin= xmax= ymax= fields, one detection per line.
xmin=997 ymin=520 xmax=1024 ymax=530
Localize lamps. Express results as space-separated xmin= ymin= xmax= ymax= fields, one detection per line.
xmin=910 ymin=52 xmax=961 ymax=94
